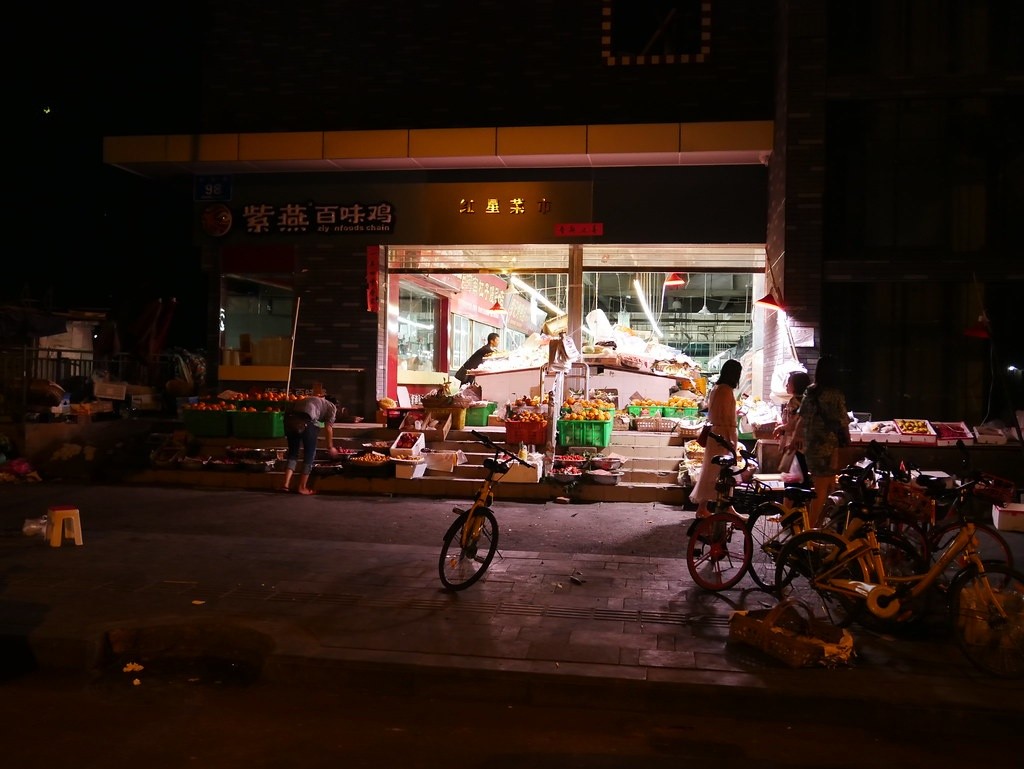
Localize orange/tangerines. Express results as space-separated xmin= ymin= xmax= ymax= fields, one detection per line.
xmin=634 ymin=396 xmax=699 ymax=407
xmin=562 ymin=398 xmax=614 ymax=420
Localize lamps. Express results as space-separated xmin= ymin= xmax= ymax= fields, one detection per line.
xmin=697 ymin=274 xmax=713 ymax=314
xmin=756 ymin=288 xmax=778 ymax=309
xmin=490 ymin=297 xmax=507 ymax=313
xmin=666 ymin=274 xmax=684 ymax=285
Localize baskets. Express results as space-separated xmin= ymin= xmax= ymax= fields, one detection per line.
xmin=724 ymin=598 xmax=853 ymax=667
xmin=735 ymin=480 xmax=773 ymax=513
xmin=880 ymin=479 xmax=928 ymax=516
xmin=973 ymin=474 xmax=1017 ymax=507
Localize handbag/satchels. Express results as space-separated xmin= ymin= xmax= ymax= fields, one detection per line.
xmin=283 ymin=414 xmax=308 ymax=434
xmin=835 ymin=422 xmax=848 ymax=447
xmin=454 ymin=366 xmax=470 ymax=383
xmin=697 ymin=425 xmax=713 ymax=447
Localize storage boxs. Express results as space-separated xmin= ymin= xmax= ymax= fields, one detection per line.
xmin=626 ymin=405 xmax=698 ymax=417
xmin=558 ymin=408 xmax=615 ymax=448
xmin=504 ymin=416 xmax=548 ymax=445
xmin=506 ymin=404 xmax=535 ymax=412
xmin=849 ymin=418 xmax=1007 ymax=447
xmin=181 ymin=398 xmax=290 ymax=440
xmin=490 ymin=454 xmax=545 ymax=483
xmin=399 ymin=411 xmax=453 ymax=441
xmin=543 ymin=312 xmax=568 ymax=336
xmin=376 ymin=393 xmax=507 ymax=429
xmin=855 ymin=467 xmax=1024 ymax=532
xmin=313 ymin=432 xmax=463 ymax=478
xmin=738 ymin=415 xmax=778 ymax=440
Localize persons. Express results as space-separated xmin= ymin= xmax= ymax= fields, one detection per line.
xmin=779 ymin=355 xmax=851 ymax=528
xmin=773 ymin=371 xmax=809 ymax=475
xmin=274 ymin=396 xmax=337 ymax=497
xmin=689 ymin=360 xmax=752 ymax=522
xmin=455 ymin=333 xmax=500 ymax=388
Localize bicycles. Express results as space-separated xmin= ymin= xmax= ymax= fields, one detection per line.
xmin=440 ymin=430 xmax=535 ymax=592
xmin=688 ymin=431 xmax=1024 ymax=676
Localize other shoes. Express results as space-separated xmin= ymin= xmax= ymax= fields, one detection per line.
xmin=282 ymin=488 xmax=289 ymax=492
xmin=298 ymin=490 xmax=318 ymax=495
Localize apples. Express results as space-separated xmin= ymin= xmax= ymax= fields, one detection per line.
xmin=518 ymin=410 xmax=541 ymax=422
xmin=185 ymin=391 xmax=320 ymax=412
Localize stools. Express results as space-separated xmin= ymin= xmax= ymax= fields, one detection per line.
xmin=45 ymin=504 xmax=83 ymax=547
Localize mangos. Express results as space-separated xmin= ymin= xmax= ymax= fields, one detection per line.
xmin=896 ymin=420 xmax=927 ymax=432
